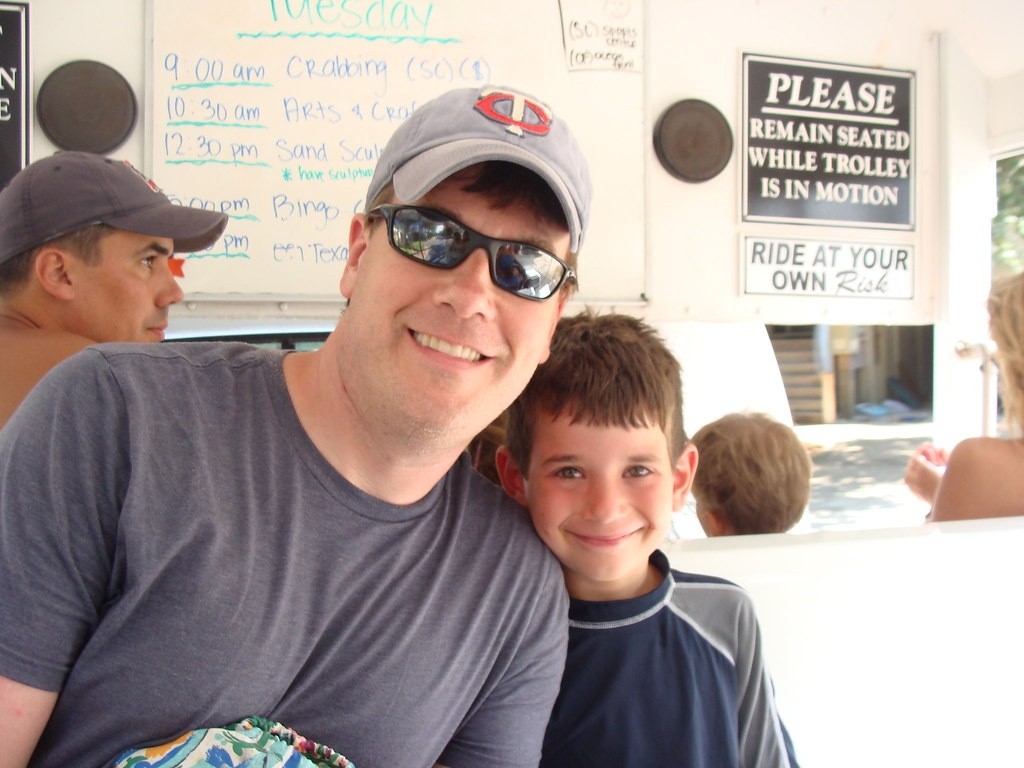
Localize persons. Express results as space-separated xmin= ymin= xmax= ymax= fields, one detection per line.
xmin=903 ymin=270 xmax=1024 ymax=523
xmin=689 ymin=412 xmax=810 ymax=538
xmin=495 ymin=243 xmax=525 ymax=290
xmin=0 ymin=85 xmax=592 ymax=768
xmin=447 ymin=231 xmax=469 ymax=266
xmin=494 ymin=313 xmax=799 ymax=768
xmin=0 ymin=149 xmax=229 ymax=429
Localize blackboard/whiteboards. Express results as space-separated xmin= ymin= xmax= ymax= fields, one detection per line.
xmin=140 ymin=1 xmax=657 ymax=315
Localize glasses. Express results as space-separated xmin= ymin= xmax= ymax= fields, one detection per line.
xmin=368 ymin=203 xmax=576 ymax=302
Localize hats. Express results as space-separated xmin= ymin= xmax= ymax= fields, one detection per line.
xmin=0 ymin=151 xmax=228 ymax=265
xmin=365 ymin=85 xmax=594 ymax=258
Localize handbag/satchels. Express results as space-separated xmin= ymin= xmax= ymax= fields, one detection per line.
xmin=111 ymin=716 xmax=355 ymax=768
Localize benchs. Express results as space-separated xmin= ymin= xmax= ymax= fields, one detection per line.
xmin=664 ymin=514 xmax=1024 ymax=768
